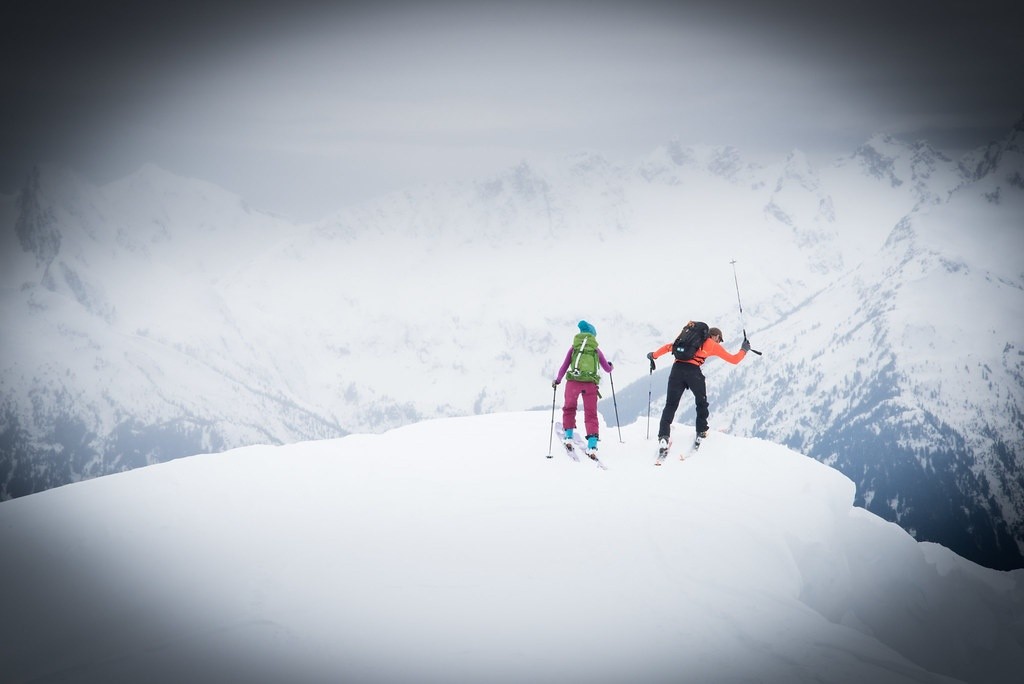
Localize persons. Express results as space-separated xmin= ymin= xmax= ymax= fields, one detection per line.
xmin=646 ymin=320 xmax=752 ymax=459
xmin=551 ymin=320 xmax=615 ymax=459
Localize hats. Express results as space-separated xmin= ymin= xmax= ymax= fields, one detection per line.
xmin=709 ymin=327 xmax=724 ymax=342
xmin=577 ymin=320 xmax=597 ymax=336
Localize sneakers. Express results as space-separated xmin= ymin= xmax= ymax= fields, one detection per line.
xmin=585 ymin=436 xmax=598 ymax=456
xmin=563 ymin=429 xmax=574 ymax=448
xmin=659 ymin=437 xmax=669 ymax=455
xmin=696 ymin=433 xmax=706 ymax=446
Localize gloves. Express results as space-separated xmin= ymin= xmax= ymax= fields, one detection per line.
xmin=742 ymin=339 xmax=750 ymax=351
xmin=552 ymin=379 xmax=559 ymax=387
xmin=608 ymin=362 xmax=612 ymax=366
xmin=646 ymin=352 xmax=658 ymax=359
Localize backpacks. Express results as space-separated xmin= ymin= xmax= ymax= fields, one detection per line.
xmin=567 ymin=333 xmax=600 ymax=384
xmin=673 ymin=321 xmax=711 ymax=361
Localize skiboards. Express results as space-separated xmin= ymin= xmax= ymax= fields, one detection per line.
xmin=654 ymin=433 xmax=711 ymax=468
xmin=555 ymin=420 xmax=609 ymax=471
